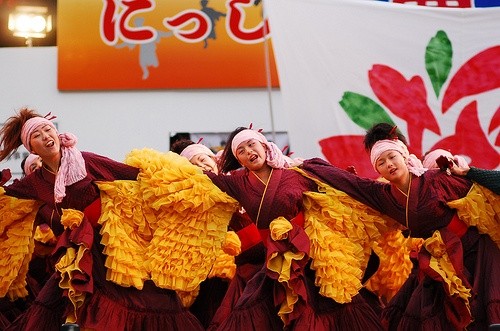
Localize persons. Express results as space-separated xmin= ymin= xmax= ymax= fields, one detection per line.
xmin=21 ymin=153 xmax=43 ymax=176
xmin=202 ymin=127 xmax=384 ymax=331
xmin=448 ymin=155 xmax=500 ymax=196
xmin=169 ymin=139 xmax=267 ymax=331
xmin=283 ymin=155 xmax=358 ymax=176
xmin=421 ymin=149 xmax=454 ymax=169
xmin=0 ymin=107 xmax=199 ymax=331
xmin=289 ymin=122 xmax=500 ymax=331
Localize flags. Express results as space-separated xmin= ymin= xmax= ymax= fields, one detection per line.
xmin=263 ymin=1 xmax=500 ymax=180
